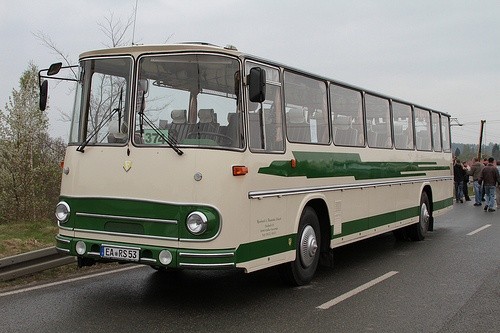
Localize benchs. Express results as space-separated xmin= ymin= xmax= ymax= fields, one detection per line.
xmin=160 ymin=107 xmax=220 ymax=148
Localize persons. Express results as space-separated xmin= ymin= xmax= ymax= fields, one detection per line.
xmin=453 ymin=159 xmax=466 ymax=203
xmin=465 ymin=157 xmax=484 ymax=207
xmin=481 ymin=157 xmax=489 ymax=202
xmin=494 ymin=160 xmax=500 ymax=209
xmin=477 ymin=157 xmax=500 ymax=212
xmin=462 ymin=161 xmax=472 ymax=201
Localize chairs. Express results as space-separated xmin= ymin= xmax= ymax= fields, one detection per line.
xmin=333 ymin=116 xmax=358 ymax=148
xmin=369 ymin=121 xmax=438 ymax=149
xmin=226 ymin=107 xmax=272 ymax=150
xmin=287 ymin=108 xmax=312 ymax=143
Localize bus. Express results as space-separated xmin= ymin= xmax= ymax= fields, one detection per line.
xmin=38 ymin=44 xmax=454 ymax=286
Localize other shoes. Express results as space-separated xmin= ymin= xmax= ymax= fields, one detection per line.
xmin=484 ymin=205 xmax=488 ymax=210
xmin=473 ymin=202 xmax=479 ymax=206
xmin=456 ymin=198 xmax=459 ymax=203
xmin=460 ymin=198 xmax=463 ymax=203
xmin=482 ymin=198 xmax=485 ymax=201
xmin=479 ymin=202 xmax=482 ymax=206
xmin=465 ymin=197 xmax=471 ymax=201
xmin=488 ymin=208 xmax=495 ymax=212
